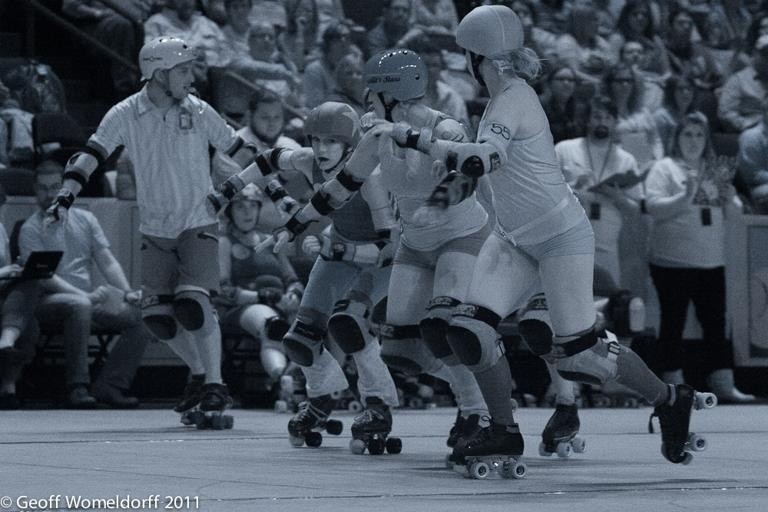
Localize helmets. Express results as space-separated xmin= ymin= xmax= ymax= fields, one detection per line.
xmin=455 ymin=6 xmax=524 ymax=60
xmin=140 ymin=37 xmax=200 ymax=80
xmin=304 ymin=101 xmax=360 ymax=150
xmin=223 ymin=182 xmax=264 ymax=215
xmin=364 ymin=49 xmax=428 ymax=101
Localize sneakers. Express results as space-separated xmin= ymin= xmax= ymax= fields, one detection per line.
xmin=89 ymin=380 xmax=139 ymax=408
xmin=68 ymin=383 xmax=97 ymax=409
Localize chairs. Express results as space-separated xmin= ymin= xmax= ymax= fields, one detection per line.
xmin=0 ymin=60 xmax=120 ymax=198
xmin=9 ymin=219 xmax=116 ymax=407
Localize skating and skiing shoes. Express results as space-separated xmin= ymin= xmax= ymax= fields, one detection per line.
xmin=174 ymin=373 xmax=233 ymax=430
xmin=393 ymin=375 xmax=434 ymax=409
xmin=540 ymin=404 xmax=585 ymax=457
xmin=655 ymin=384 xmax=717 ymax=464
xmin=447 ymin=404 xmax=527 ymax=478
xmin=288 ymin=388 xmax=360 ymax=446
xmin=350 ymin=396 xmax=401 ymax=455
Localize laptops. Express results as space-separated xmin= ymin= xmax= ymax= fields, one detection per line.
xmin=0 ymin=250 xmax=64 ymax=281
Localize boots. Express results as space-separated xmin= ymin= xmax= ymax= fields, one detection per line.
xmin=662 ymin=369 xmax=685 ymax=384
xmin=710 ymin=369 xmax=754 ymax=403
